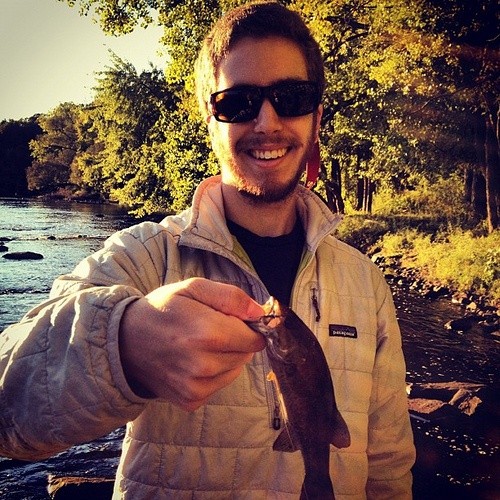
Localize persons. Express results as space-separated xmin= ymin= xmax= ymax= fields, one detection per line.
xmin=0 ymin=2 xmax=417 ymax=500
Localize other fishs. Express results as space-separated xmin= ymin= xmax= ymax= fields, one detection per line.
xmin=248 ymin=296 xmax=353 ymax=500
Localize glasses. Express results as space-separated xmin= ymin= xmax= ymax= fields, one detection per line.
xmin=210 ymin=80 xmax=324 ymax=122
